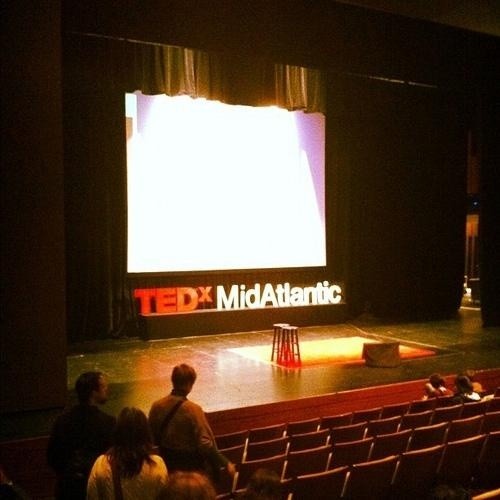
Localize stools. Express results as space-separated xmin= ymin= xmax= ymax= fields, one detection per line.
xmin=279 ymin=325 xmax=301 ymax=365
xmin=270 ymin=322 xmax=291 ymax=364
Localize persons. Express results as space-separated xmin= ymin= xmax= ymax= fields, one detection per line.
xmin=86 ymin=406 xmax=167 ymax=500
xmin=454 ymin=368 xmax=483 ymax=394
xmin=450 ymin=376 xmax=481 ymax=408
xmin=427 ymin=374 xmax=454 ymax=398
xmin=147 ymin=364 xmax=234 ymax=482
xmin=244 ymin=469 xmax=280 ymax=500
xmin=46 ymin=371 xmax=116 ymax=497
xmin=164 ymin=435 xmax=216 ymax=500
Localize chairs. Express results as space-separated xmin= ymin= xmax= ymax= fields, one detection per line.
xmin=0 ymin=362 xmax=500 ymax=500
